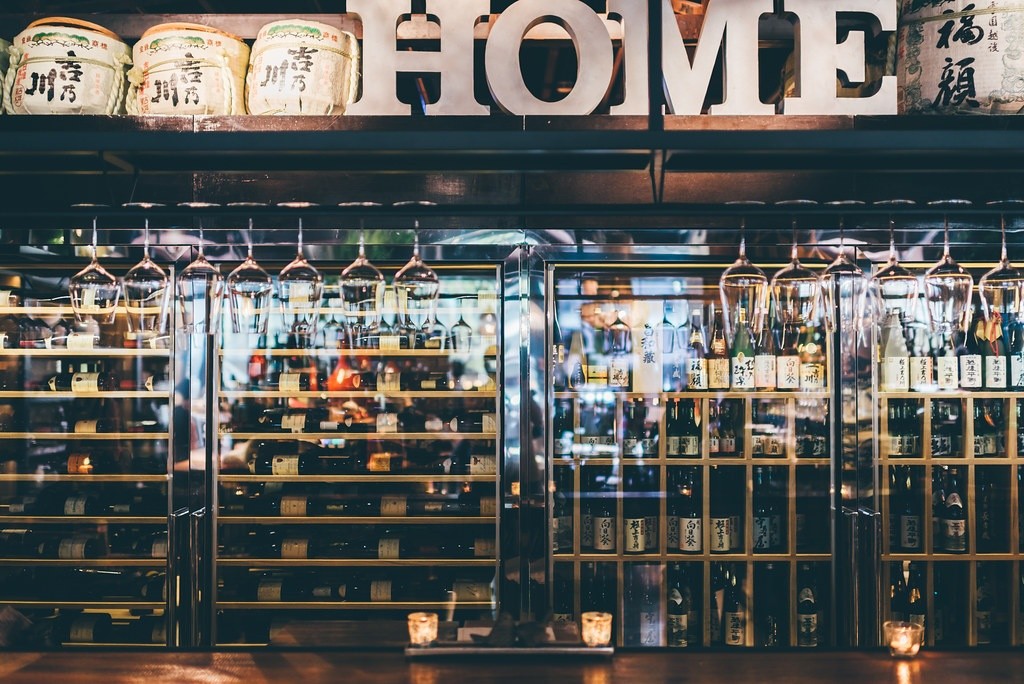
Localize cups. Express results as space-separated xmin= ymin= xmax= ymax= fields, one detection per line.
xmin=582 ymin=611 xmax=611 ymax=646
xmin=883 ymin=621 xmax=924 ymax=657
xmin=407 ymin=612 xmax=438 ymax=645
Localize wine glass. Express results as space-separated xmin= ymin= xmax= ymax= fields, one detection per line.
xmin=393 ymin=200 xmax=440 ymax=334
xmin=819 ymin=200 xmax=868 ymax=331
xmin=68 ymin=203 xmax=121 ymax=325
xmin=719 ymin=201 xmax=768 ymax=348
xmin=337 ymin=202 xmax=384 ymax=334
xmin=123 ymin=202 xmax=171 ymax=334
xmin=227 ymin=202 xmax=272 ymax=333
xmin=177 ymin=202 xmax=226 ymax=334
xmin=923 ymin=199 xmax=975 ymax=332
xmin=869 ymin=199 xmax=919 ymax=325
xmin=771 ymin=199 xmax=820 ymax=326
xmin=277 ymin=203 xmax=322 ymax=334
xmin=604 ymin=276 xmax=630 ymax=353
xmin=978 ymin=199 xmax=1024 ymax=322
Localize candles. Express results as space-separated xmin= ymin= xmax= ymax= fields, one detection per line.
xmin=883 ymin=620 xmax=925 ymax=659
xmin=407 ymin=612 xmax=439 ymax=648
xmin=580 ymin=612 xmax=614 ymax=647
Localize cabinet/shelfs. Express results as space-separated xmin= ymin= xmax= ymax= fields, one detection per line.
xmin=0 ymin=257 xmax=1024 ymax=655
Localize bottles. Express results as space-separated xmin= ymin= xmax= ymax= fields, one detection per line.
xmin=0 ymin=529 xmax=168 ymax=643
xmin=216 ymin=452 xmax=498 ymax=559
xmin=684 ymin=309 xmax=825 ymax=392
xmin=220 ymin=407 xmax=496 ymax=433
xmin=711 ymin=562 xmax=828 ymax=648
xmin=217 ymin=579 xmax=496 ymax=602
xmin=753 ymin=400 xmax=828 ymax=458
xmin=552 ymin=306 xmax=632 ymax=393
xmin=555 ymin=561 xmax=701 ymax=646
xmin=0 ymin=287 xmax=170 ymax=350
xmin=219 ymin=331 xmax=498 ymax=391
xmin=890 ymin=464 xmax=1003 ymax=554
xmin=1 ymin=413 xmax=168 ymax=517
xmin=665 ymin=399 xmax=737 ymax=457
xmin=0 ymin=363 xmax=169 ymax=392
xmin=553 ymin=466 xmax=660 ymax=555
xmin=551 ymin=398 xmax=574 ymax=459
xmin=580 ymin=398 xmax=660 ymax=458
xmin=666 ymin=466 xmax=826 ymax=555
xmin=888 ymin=399 xmax=1024 ymax=458
xmin=891 ymin=561 xmax=1008 ymax=646
xmin=880 ymin=306 xmax=1024 ymax=392
